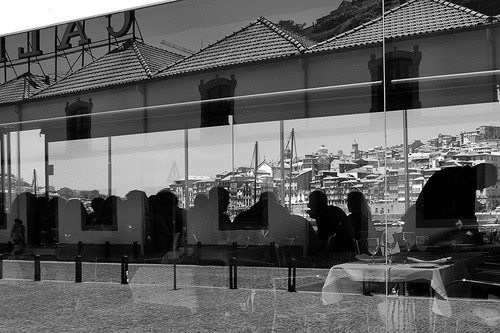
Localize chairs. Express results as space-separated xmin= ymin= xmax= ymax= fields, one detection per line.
xmin=432 ymin=262 xmax=500 ymax=333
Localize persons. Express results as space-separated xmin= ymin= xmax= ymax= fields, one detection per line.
xmin=415 ymin=163 xmax=497 ymax=246
xmin=0 ymin=188 xmax=320 ymax=268
xmin=306 ymin=190 xmax=348 ymax=269
xmin=347 ymin=192 xmax=382 ymax=257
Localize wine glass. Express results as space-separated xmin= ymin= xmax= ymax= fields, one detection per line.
xmin=401 ymin=232 xmax=416 ymax=263
xmin=385 ymin=232 xmax=397 ymax=266
xmin=366 ymin=238 xmax=380 ymax=266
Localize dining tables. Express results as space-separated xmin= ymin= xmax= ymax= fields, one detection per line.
xmin=321 ymin=252 xmax=487 ymax=333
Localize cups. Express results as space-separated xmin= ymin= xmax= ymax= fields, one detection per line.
xmin=416 ymin=236 xmax=428 ymax=251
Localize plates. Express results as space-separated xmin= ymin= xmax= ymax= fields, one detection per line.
xmin=356 ymin=255 xmax=383 ymax=262
